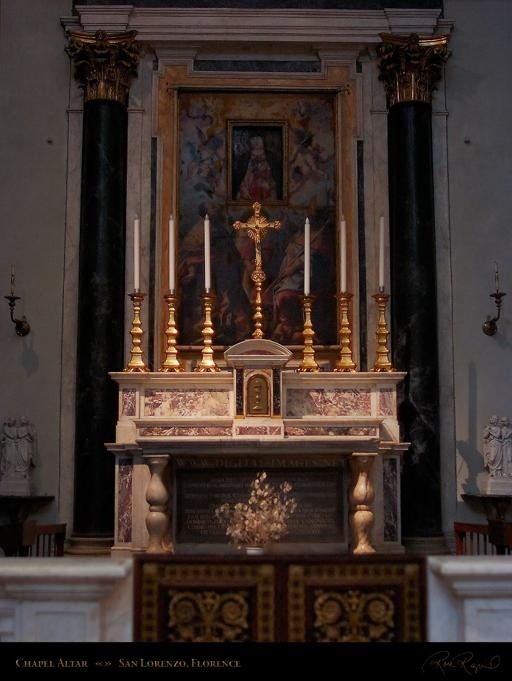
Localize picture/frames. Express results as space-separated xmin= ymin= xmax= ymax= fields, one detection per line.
xmin=158 ymin=73 xmax=354 ymax=366
xmin=226 ymin=117 xmax=290 ymax=207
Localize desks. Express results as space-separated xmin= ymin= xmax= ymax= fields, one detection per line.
xmin=461 ymin=495 xmax=509 ymax=556
xmin=0 ymin=494 xmax=56 ymax=557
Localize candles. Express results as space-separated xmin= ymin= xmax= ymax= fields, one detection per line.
xmin=495 ymin=263 xmax=499 ymax=291
xmin=133 ymin=213 xmax=210 ymax=290
xmin=304 ymin=215 xmax=385 ymax=295
xmin=10 ymin=263 xmax=14 ymax=296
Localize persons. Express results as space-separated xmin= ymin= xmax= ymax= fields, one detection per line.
xmin=496 ymin=415 xmax=511 ymax=478
xmin=236 ymin=133 xmax=279 ymax=200
xmin=0 ymin=415 xmax=19 ymax=481
xmin=481 ymin=413 xmax=503 ymax=479
xmin=13 ymin=415 xmax=41 ymax=482
